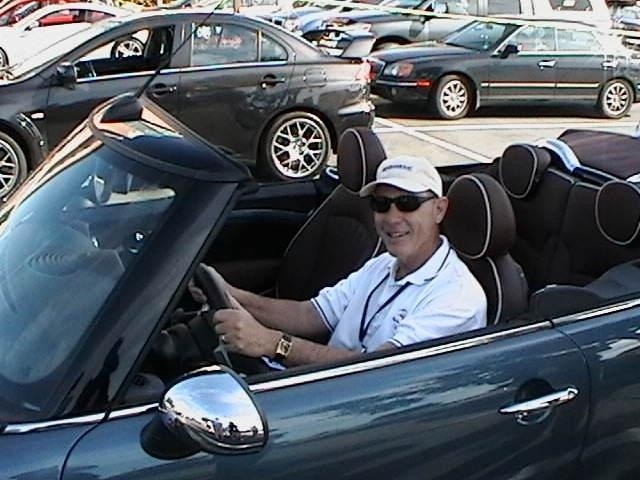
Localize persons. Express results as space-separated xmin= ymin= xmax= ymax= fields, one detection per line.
xmin=188 ymin=155 xmax=489 ymax=367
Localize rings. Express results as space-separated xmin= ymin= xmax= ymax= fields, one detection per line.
xmin=221 ymin=334 xmax=225 ymax=342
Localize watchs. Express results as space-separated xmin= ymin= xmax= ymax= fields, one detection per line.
xmin=268 ymin=333 xmax=296 ymax=362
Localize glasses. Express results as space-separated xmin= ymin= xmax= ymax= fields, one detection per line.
xmin=371 ymin=193 xmax=438 ymax=213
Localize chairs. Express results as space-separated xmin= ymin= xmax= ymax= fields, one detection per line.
xmin=491 ymin=143 xmax=640 ymax=333
xmin=441 ymin=172 xmax=529 ymax=334
xmin=261 ymin=126 xmax=400 ymax=322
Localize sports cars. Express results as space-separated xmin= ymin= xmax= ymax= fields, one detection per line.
xmin=0 ymin=91 xmax=640 ymax=480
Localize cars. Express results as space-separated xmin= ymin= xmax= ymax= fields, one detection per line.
xmin=361 ymin=16 xmax=640 ymax=121
xmin=1 ymin=10 xmax=376 ymax=207
xmin=0 ymin=0 xmax=640 ymax=70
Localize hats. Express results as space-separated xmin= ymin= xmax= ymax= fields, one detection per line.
xmin=359 ymin=154 xmax=443 ymax=200
